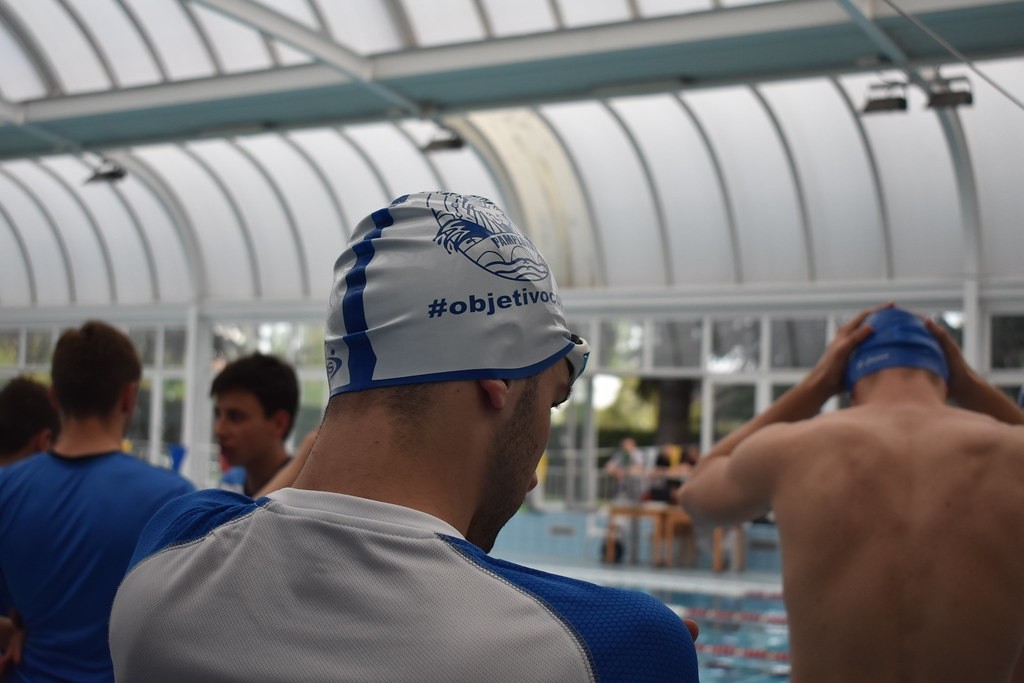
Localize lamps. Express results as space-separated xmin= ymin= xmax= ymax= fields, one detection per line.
xmin=85 ymin=151 xmax=125 ymax=182
xmin=415 ymin=100 xmax=464 ymax=150
xmin=925 ymin=77 xmax=972 ymax=106
xmin=861 ymin=83 xmax=908 ymax=114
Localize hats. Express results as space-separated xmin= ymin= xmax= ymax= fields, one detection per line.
xmin=846 ymin=308 xmax=949 ymax=392
xmin=323 ymin=191 xmax=574 ymax=405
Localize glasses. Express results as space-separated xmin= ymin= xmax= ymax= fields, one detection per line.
xmin=566 ymin=336 xmax=591 ymax=386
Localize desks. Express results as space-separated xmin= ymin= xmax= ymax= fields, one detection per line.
xmin=605 ymin=502 xmax=744 ymax=572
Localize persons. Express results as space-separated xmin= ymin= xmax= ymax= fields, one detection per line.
xmin=678 ymin=301 xmax=1024 ymax=683
xmin=0 ymin=192 xmax=706 ymax=683
